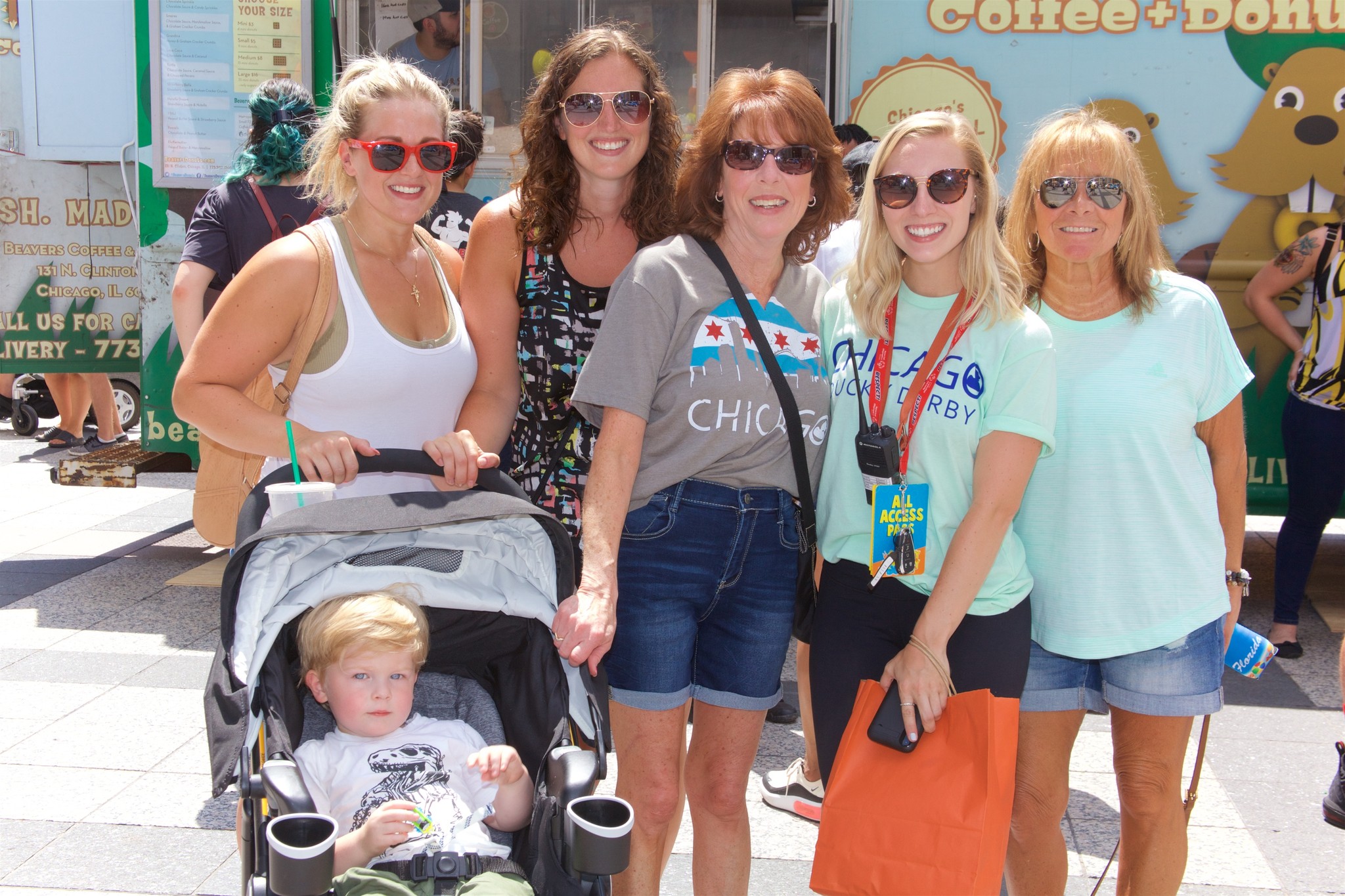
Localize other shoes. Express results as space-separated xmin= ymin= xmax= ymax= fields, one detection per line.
xmin=767 ymin=698 xmax=799 ymax=724
xmin=1267 ymin=628 xmax=1303 ymax=658
xmin=1322 ymin=741 xmax=1345 ymax=827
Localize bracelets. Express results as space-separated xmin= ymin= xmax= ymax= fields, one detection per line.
xmin=1225 ymin=569 xmax=1252 ymax=598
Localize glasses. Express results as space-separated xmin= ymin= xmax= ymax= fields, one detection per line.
xmin=722 ymin=140 xmax=817 ymax=175
xmin=1037 ymin=176 xmax=1126 ymax=211
xmin=559 ymin=90 xmax=655 ymax=127
xmin=347 ymin=138 xmax=458 ymax=173
xmin=873 ymin=169 xmax=979 ymax=210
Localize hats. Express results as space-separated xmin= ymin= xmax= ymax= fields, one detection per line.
xmin=843 ymin=139 xmax=879 ymax=191
xmin=408 ymin=0 xmax=471 ymax=23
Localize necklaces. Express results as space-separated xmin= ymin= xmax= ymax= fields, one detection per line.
xmin=342 ymin=208 xmax=422 ymax=307
xmin=1294 ymin=348 xmax=1302 ymax=354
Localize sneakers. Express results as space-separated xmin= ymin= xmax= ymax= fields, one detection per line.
xmin=115 ymin=432 xmax=129 ymax=442
xmin=67 ymin=434 xmax=119 ymax=456
xmin=762 ymin=757 xmax=825 ymax=821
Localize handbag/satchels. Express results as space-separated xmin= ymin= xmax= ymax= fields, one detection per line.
xmin=792 ymin=508 xmax=818 ymax=648
xmin=190 ymin=226 xmax=332 ymax=548
xmin=810 ymin=636 xmax=1020 ymax=896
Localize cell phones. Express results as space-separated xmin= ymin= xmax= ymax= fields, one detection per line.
xmin=867 ymin=681 xmax=924 ymax=753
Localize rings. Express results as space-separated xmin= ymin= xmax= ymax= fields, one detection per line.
xmin=900 ymin=702 xmax=916 ymax=707
xmin=554 ymin=631 xmax=564 ymax=642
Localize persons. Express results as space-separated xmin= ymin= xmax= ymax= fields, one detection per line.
xmin=289 ymin=591 xmax=537 ymax=896
xmin=0 ymin=373 xmax=16 ymax=417
xmin=758 ymin=118 xmax=877 ymax=828
xmin=1243 ymin=220 xmax=1345 ymax=660
xmin=424 ymin=29 xmax=688 ymax=801
xmin=34 ymin=373 xmax=92 ymax=448
xmin=170 ymin=57 xmax=477 ymax=495
xmin=542 ymin=63 xmax=853 ymax=896
xmin=414 ymin=108 xmax=491 ymax=261
xmin=168 ymin=77 xmax=346 ymax=367
xmin=1003 ymin=113 xmax=1255 ymax=896
xmin=68 ymin=373 xmax=130 ymax=456
xmin=812 ymin=110 xmax=1055 ymax=896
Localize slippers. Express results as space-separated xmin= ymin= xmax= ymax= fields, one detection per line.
xmin=34 ymin=426 xmax=62 ymax=442
xmin=49 ymin=430 xmax=86 ymax=448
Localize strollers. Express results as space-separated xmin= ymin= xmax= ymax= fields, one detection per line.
xmin=193 ymin=444 xmax=634 ymax=895
